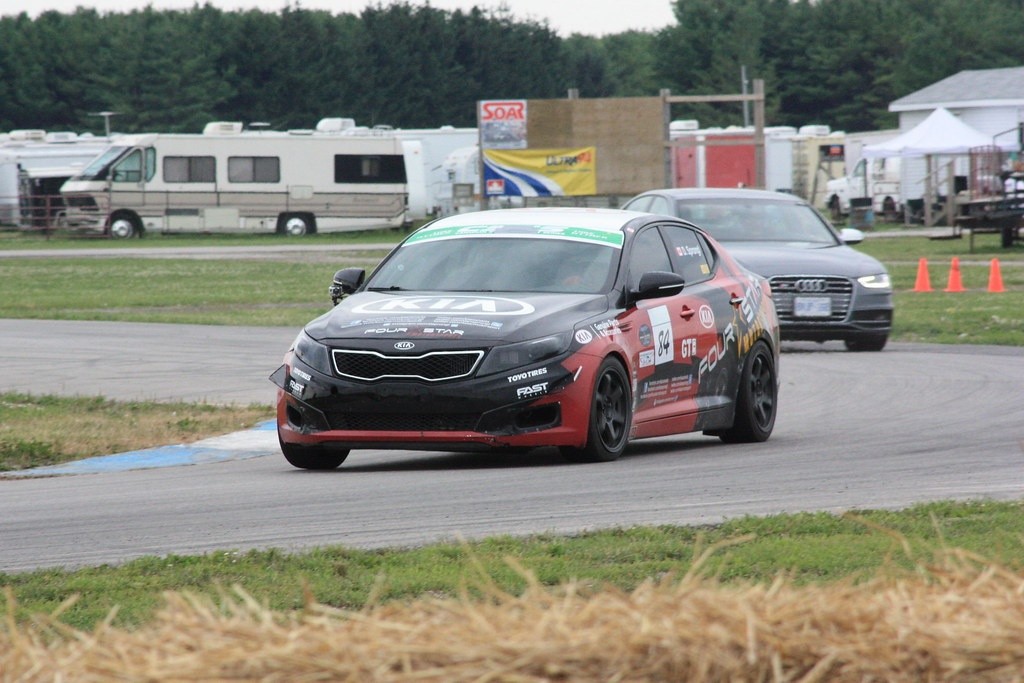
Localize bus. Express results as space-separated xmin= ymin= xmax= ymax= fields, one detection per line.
xmin=58 ymin=117 xmax=410 ymax=240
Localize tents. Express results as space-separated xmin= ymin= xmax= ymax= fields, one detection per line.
xmin=862 ymin=108 xmax=1021 ymax=229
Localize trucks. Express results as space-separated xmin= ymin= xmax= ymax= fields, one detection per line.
xmin=823 ymin=155 xmax=903 ymax=218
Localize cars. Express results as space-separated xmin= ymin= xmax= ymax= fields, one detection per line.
xmin=268 ymin=206 xmax=781 ymax=469
xmin=618 ymin=187 xmax=894 ymax=351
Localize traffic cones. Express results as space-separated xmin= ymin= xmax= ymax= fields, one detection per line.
xmin=912 ymin=254 xmax=933 ymax=292
xmin=983 ymin=259 xmax=1006 ymax=292
xmin=941 ymin=257 xmax=964 ymax=292
